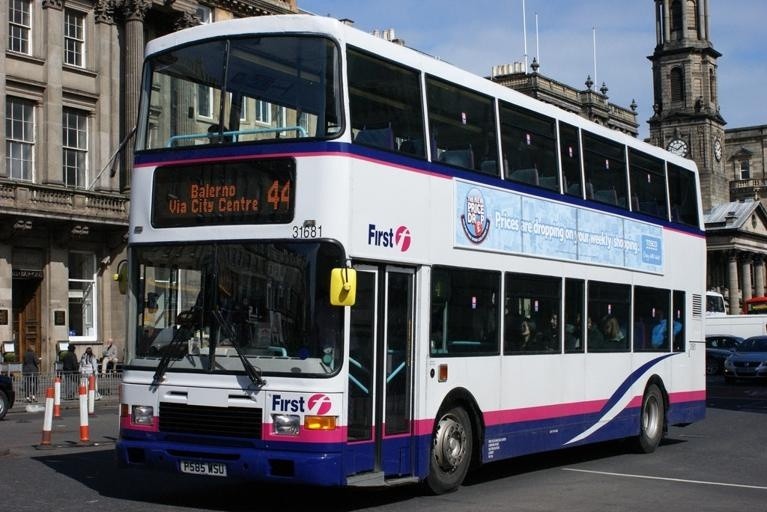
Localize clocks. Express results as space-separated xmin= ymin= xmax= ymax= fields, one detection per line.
xmin=666 ymin=138 xmax=689 ymax=159
xmin=713 ymin=139 xmax=723 ymax=162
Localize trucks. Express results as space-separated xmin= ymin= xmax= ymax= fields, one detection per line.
xmin=705 ymin=292 xmax=767 ymax=339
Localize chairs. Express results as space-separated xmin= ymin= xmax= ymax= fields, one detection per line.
xmin=353 ymin=117 xmax=643 ymax=215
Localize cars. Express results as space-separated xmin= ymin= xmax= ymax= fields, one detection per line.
xmin=706 ymin=336 xmax=767 ymax=385
xmin=0 ymin=375 xmax=15 ymax=420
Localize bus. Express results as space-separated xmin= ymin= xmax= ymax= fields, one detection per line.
xmin=113 ymin=13 xmax=707 ymax=495
xmin=744 ymin=297 xmax=767 ymax=314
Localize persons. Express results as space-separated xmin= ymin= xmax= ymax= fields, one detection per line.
xmin=480 ymin=309 xmax=684 ymax=351
xmin=22 ymin=343 xmax=43 ymax=402
xmin=208 ymin=124 xmax=231 ymax=144
xmin=101 ymin=338 xmax=120 ymax=379
xmin=62 ymin=343 xmax=98 ymax=399
